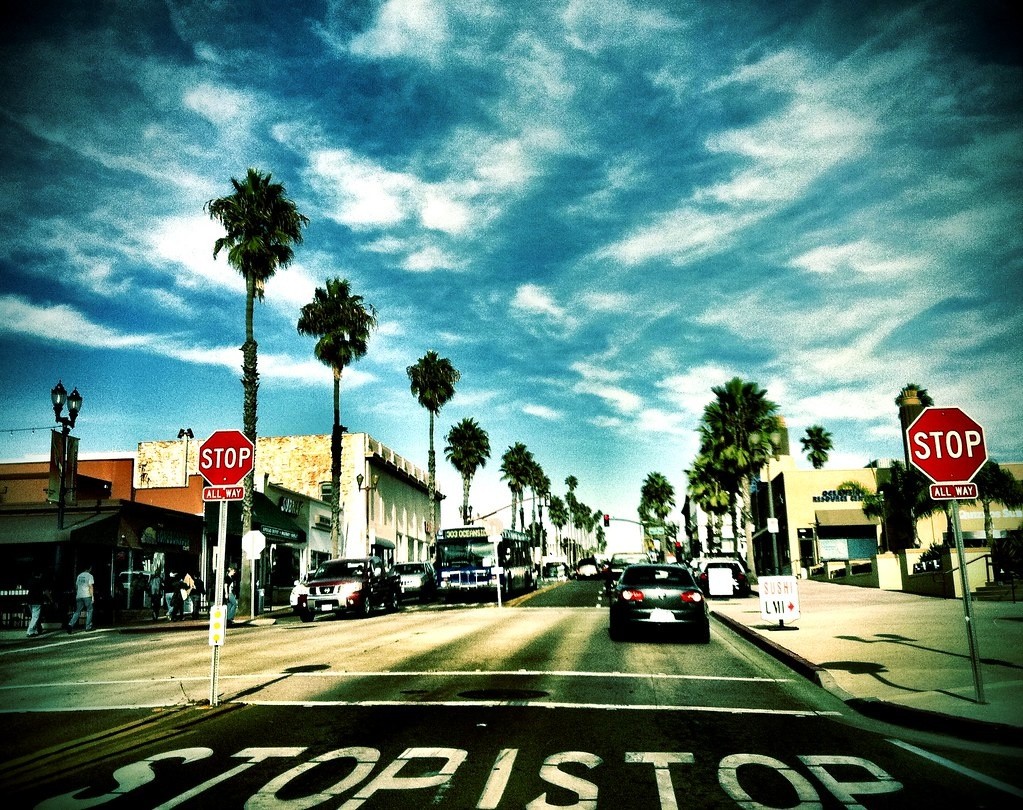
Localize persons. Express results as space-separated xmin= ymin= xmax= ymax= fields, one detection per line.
xmin=352 ymin=565 xmax=364 ymax=575
xmin=224 ymin=568 xmax=236 ymax=624
xmin=26 ymin=570 xmax=54 ymax=638
xmin=66 ymin=563 xmax=95 ymax=635
xmin=114 ymin=567 xmax=206 ymax=622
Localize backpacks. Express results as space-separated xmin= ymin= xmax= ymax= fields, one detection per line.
xmin=170 ymin=590 xmax=182 ymax=605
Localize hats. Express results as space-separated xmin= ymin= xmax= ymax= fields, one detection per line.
xmin=180 ymin=583 xmax=190 ymax=590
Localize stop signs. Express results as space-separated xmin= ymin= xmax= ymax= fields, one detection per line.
xmin=906 ymin=406 xmax=989 ymax=484
xmin=197 ymin=429 xmax=255 ymax=489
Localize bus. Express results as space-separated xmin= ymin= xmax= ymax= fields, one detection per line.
xmin=429 ymin=524 xmax=538 ymax=603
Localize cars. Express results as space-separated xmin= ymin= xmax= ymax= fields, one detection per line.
xmin=697 ymin=557 xmax=752 ymax=598
xmin=608 ymin=563 xmax=711 ymax=645
xmin=390 ymin=561 xmax=435 ymax=602
xmin=545 ymin=556 xmax=607 ymax=580
xmin=290 ymin=570 xmax=317 ymax=616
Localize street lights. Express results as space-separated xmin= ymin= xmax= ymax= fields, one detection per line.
xmin=355 ymin=472 xmax=382 ymax=555
xmin=747 ymin=429 xmax=785 ymax=629
xmin=459 ymin=505 xmax=472 ymax=525
xmin=50 ymin=380 xmax=83 ymax=580
xmin=177 ymin=428 xmax=195 ymax=488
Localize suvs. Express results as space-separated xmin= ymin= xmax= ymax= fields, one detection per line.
xmin=303 ymin=557 xmax=402 ymax=617
xmin=603 ymin=551 xmax=653 ymax=595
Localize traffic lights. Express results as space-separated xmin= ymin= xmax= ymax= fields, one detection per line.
xmin=603 ymin=515 xmax=610 ymax=526
xmin=675 ymin=542 xmax=682 ymax=555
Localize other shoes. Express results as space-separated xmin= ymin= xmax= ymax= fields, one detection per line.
xmin=40 ymin=629 xmax=47 ymax=634
xmin=172 ymin=617 xmax=178 ymax=622
xmin=27 ymin=631 xmax=38 ymax=637
xmin=65 ymin=625 xmax=73 ymax=634
xmin=180 ymin=617 xmax=186 ymax=621
